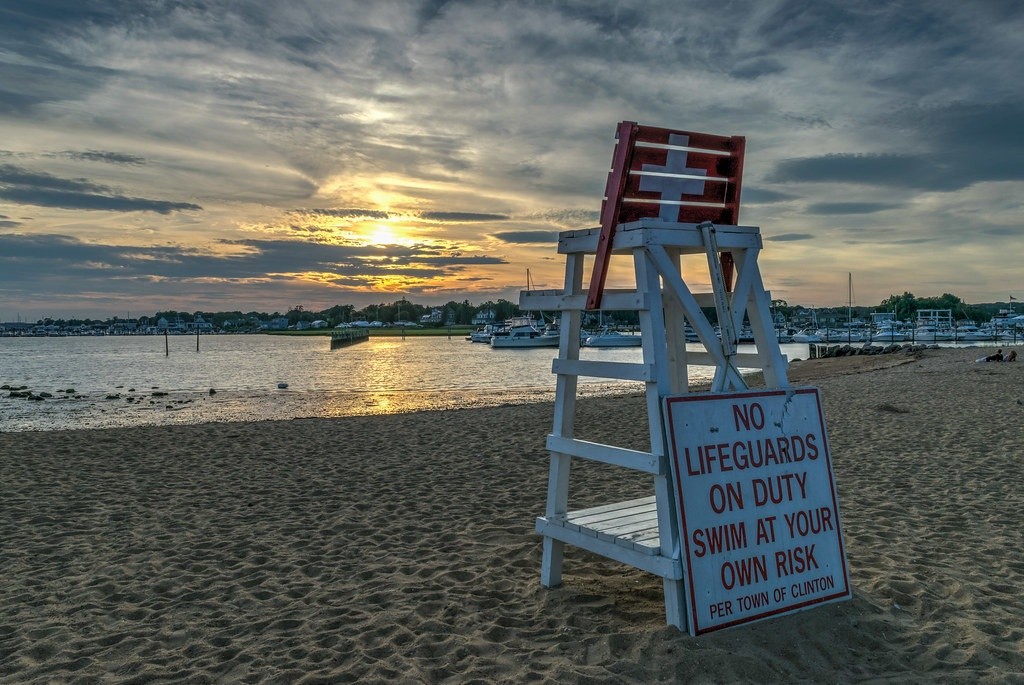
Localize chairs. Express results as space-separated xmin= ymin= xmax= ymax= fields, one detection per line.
xmin=535 ymin=122 xmax=790 ymax=632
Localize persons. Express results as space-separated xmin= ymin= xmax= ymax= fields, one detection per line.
xmin=975 ymin=349 xmax=1003 ymax=362
xmin=990 ymin=351 xmax=1017 ymax=362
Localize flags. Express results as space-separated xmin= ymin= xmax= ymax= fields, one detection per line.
xmin=1010 ymin=295 xmax=1018 ymax=300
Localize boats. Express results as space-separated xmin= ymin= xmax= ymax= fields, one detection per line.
xmin=680 ymin=273 xmax=1024 ymax=344
xmin=470 ymin=268 xmax=642 ymax=348
